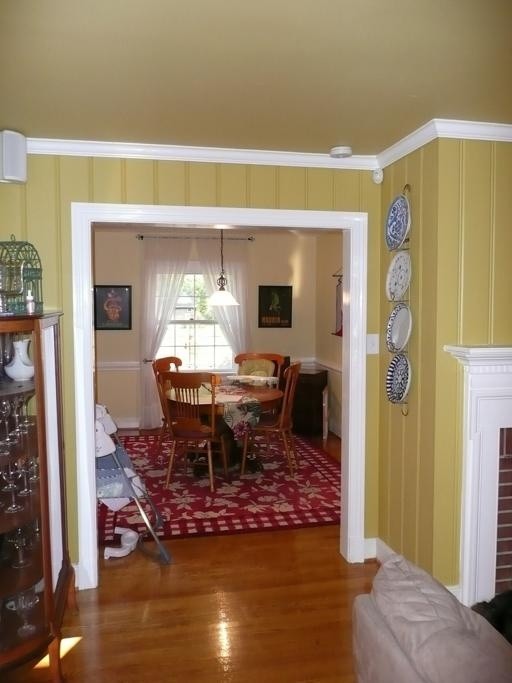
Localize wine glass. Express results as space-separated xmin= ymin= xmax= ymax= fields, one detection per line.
xmin=3 ymin=454 xmax=38 ymax=514
xmin=22 ymin=392 xmax=35 ymax=429
xmin=0 ymin=400 xmax=19 ymax=447
xmin=10 ymin=397 xmax=28 ymax=438
xmin=14 ymin=586 xmax=37 ymax=638
xmin=2 ymin=517 xmax=41 ymax=570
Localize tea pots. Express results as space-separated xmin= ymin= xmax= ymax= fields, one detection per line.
xmin=3 ymin=338 xmax=35 ymax=381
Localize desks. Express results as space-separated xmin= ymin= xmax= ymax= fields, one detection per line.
xmin=164 ymin=384 xmax=284 ymax=466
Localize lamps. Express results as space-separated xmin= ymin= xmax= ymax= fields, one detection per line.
xmin=205 ymin=235 xmax=238 ymax=308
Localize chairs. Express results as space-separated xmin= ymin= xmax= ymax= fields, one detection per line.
xmin=151 ymin=357 xmax=181 ymax=452
xmin=239 ymin=361 xmax=302 ymax=477
xmin=235 ymin=354 xmax=284 ymax=389
xmin=157 ymin=371 xmax=231 ymax=493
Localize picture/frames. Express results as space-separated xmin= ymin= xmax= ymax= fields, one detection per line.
xmin=94 ymin=285 xmax=132 ymax=331
xmin=257 ymin=284 xmax=293 ymax=329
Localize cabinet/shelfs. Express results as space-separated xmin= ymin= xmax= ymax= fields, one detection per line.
xmin=1 ymin=313 xmax=77 ymax=683
xmin=293 ymin=369 xmax=329 ymax=433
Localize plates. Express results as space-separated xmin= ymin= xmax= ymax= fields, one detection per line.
xmin=226 ymin=376 xmax=279 ymax=387
xmin=385 ymin=303 xmax=412 ymax=353
xmin=385 ymin=355 xmax=411 ymax=403
xmin=385 ymin=252 xmax=412 ymax=301
xmin=385 ymin=194 xmax=411 ymax=250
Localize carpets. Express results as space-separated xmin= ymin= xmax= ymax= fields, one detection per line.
xmin=99 ymin=431 xmax=341 ymax=546
